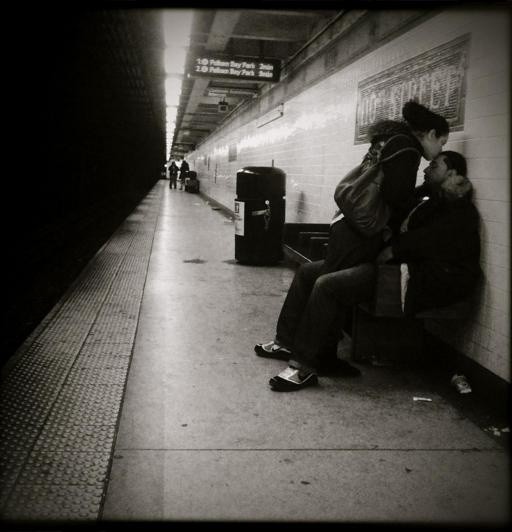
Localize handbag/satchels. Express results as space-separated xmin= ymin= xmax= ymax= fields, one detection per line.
xmin=334 ymin=132 xmax=423 ymax=242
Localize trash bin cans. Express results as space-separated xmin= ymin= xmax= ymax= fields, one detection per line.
xmin=233 ymin=166 xmax=286 ymax=266
xmin=184 ymin=171 xmax=197 ymax=193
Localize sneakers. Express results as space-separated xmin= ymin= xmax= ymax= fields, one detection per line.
xmin=268 ymin=360 xmax=319 ymax=391
xmin=320 ymin=358 xmax=363 ymax=379
xmin=252 ymin=338 xmax=294 ymax=361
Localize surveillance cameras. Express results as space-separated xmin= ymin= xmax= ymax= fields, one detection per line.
xmin=218 ymin=101 xmax=228 ymax=113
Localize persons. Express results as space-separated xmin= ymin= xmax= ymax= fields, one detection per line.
xmin=179 ymin=161 xmax=189 ymax=190
xmin=169 ymin=162 xmax=179 ymax=189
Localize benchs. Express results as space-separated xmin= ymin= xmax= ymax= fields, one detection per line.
xmin=280 ymin=220 xmax=434 ymax=381
